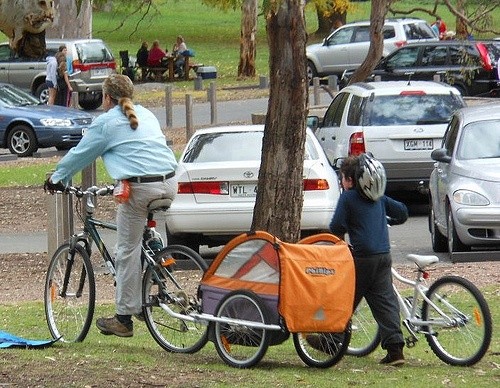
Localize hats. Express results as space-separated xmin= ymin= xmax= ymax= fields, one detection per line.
xmin=436 ymin=16 xmax=441 ymax=22
xmin=102 ymin=75 xmax=134 ymax=100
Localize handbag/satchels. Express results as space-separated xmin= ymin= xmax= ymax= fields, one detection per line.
xmin=178 ymin=49 xmax=193 ymax=56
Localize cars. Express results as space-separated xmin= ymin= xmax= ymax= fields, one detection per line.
xmin=0 ymin=83 xmax=96 ymax=158
xmin=165 ymin=123 xmax=347 ymax=261
xmin=306 ymin=79 xmax=466 ymax=197
xmin=428 ymin=102 xmax=500 ymax=256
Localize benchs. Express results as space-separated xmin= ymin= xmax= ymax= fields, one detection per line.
xmin=131 ymin=65 xmax=166 ymax=80
xmin=180 ymin=62 xmax=204 ymax=76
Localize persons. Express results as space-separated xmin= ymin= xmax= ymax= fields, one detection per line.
xmin=322 ymin=152 xmax=404 ymax=366
xmin=47 ymin=74 xmax=178 ymax=338
xmin=431 ymin=14 xmax=454 ymax=41
xmin=46 ymin=44 xmax=73 ymax=107
xmin=136 ymin=35 xmax=187 ymax=81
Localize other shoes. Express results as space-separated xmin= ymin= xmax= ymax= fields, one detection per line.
xmin=179 ymin=72 xmax=185 ymax=78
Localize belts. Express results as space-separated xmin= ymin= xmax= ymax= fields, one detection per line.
xmin=127 ymin=171 xmax=175 ymax=183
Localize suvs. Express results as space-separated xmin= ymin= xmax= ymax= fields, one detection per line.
xmin=306 ymin=17 xmax=440 ymax=86
xmin=0 ymin=39 xmax=117 ymax=111
xmin=339 ymin=38 xmax=500 ymax=97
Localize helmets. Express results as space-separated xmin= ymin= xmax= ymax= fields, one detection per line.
xmin=356 ymin=152 xmax=386 ymax=201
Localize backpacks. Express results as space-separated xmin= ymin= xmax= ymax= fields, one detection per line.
xmin=122 ymin=66 xmax=138 ymax=82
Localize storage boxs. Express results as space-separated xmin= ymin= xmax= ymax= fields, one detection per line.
xmin=195 ymin=66 xmax=217 ymax=78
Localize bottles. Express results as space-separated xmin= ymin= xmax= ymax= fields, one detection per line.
xmin=148 ymin=221 xmax=164 ymax=246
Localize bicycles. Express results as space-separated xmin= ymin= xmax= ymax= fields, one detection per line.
xmin=301 ymin=253 xmax=494 ymax=372
xmin=43 ymin=182 xmax=214 ymax=357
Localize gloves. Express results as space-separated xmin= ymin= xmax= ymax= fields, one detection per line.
xmin=43 ymin=177 xmax=64 ymax=195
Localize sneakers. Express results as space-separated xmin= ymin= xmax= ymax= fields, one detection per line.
xmin=96 ymin=315 xmax=134 ymax=337
xmin=306 ymin=333 xmax=341 ymax=354
xmin=153 ymin=255 xmax=174 ymax=285
xmin=380 ymin=343 xmax=405 ymax=366
